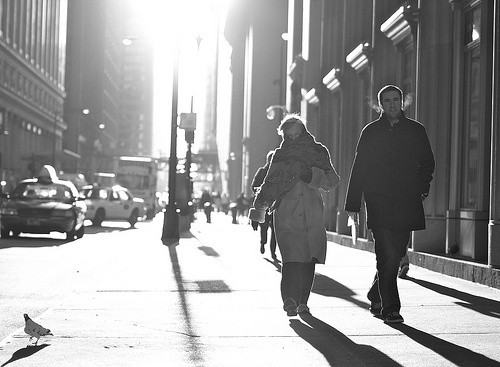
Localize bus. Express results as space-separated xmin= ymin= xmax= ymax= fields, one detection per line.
xmin=116 ymin=155 xmax=158 ymax=221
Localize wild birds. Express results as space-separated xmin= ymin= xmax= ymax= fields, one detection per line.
xmin=22 ymin=313 xmax=53 ymax=344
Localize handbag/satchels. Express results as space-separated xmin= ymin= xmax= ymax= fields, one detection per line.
xmin=249 ymin=208 xmax=266 ymax=223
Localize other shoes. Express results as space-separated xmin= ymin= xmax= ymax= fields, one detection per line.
xmin=385 ymin=310 xmax=403 ymax=323
xmin=272 ymin=251 xmax=276 ymax=260
xmin=370 ymin=300 xmax=383 ymax=316
xmin=298 ymin=304 xmax=309 ymax=314
xmin=287 ymin=307 xmax=298 ymax=316
xmin=398 ymin=266 xmax=409 ymax=277
xmin=261 ymin=243 xmax=265 ymax=254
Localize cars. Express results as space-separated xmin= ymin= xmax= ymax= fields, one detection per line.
xmin=0 ymin=165 xmax=85 ymax=242
xmin=78 ymin=182 xmax=145 ymax=227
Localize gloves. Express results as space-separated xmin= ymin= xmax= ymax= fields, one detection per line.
xmin=300 ymin=165 xmax=311 ymax=183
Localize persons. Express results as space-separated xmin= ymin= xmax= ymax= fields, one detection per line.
xmin=248 ymin=113 xmax=340 ymax=316
xmin=345 ymin=84 xmax=435 ymax=323
xmin=250 ymin=149 xmax=276 ymax=259
xmin=54 ymin=186 xmax=66 ymax=199
xmin=198 ymin=189 xmax=255 ymax=217
